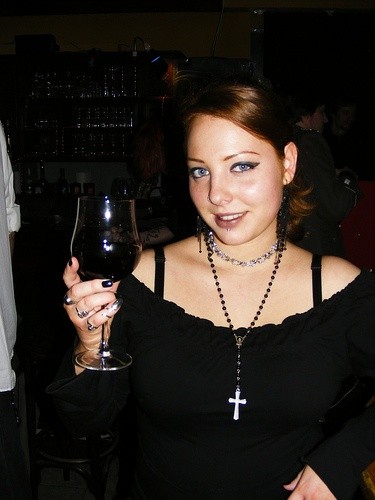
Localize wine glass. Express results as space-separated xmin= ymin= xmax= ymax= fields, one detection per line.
xmin=68 ymin=194 xmax=140 ymax=372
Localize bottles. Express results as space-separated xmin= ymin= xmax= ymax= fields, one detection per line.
xmin=6 ymin=62 xmax=140 ymax=160
xmin=58 ymin=167 xmax=69 ymax=193
xmin=38 ymin=165 xmax=50 ymax=195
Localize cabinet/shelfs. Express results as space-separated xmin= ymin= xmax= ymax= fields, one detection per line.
xmin=21 ymin=50 xmax=182 ymax=226
xmin=0 ymin=54 xmax=22 ymax=199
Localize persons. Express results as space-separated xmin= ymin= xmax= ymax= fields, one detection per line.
xmin=0 ymin=121 xmax=37 ymax=500
xmin=280 ymin=88 xmax=363 ymax=262
xmin=45 ymin=83 xmax=375 ymax=500
xmin=322 ymin=90 xmax=374 ymax=181
xmin=109 ymin=130 xmax=202 ymax=253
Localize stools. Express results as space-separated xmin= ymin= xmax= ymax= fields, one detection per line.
xmin=32 ymin=415 xmax=119 ymax=500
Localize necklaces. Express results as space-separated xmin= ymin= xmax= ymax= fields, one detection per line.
xmin=201 ymin=224 xmax=286 ymax=420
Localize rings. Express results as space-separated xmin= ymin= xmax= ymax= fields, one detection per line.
xmin=76 ymin=305 xmax=90 ymax=320
xmin=86 ymin=319 xmax=99 ymax=331
xmin=63 ymin=293 xmax=75 ymax=306
